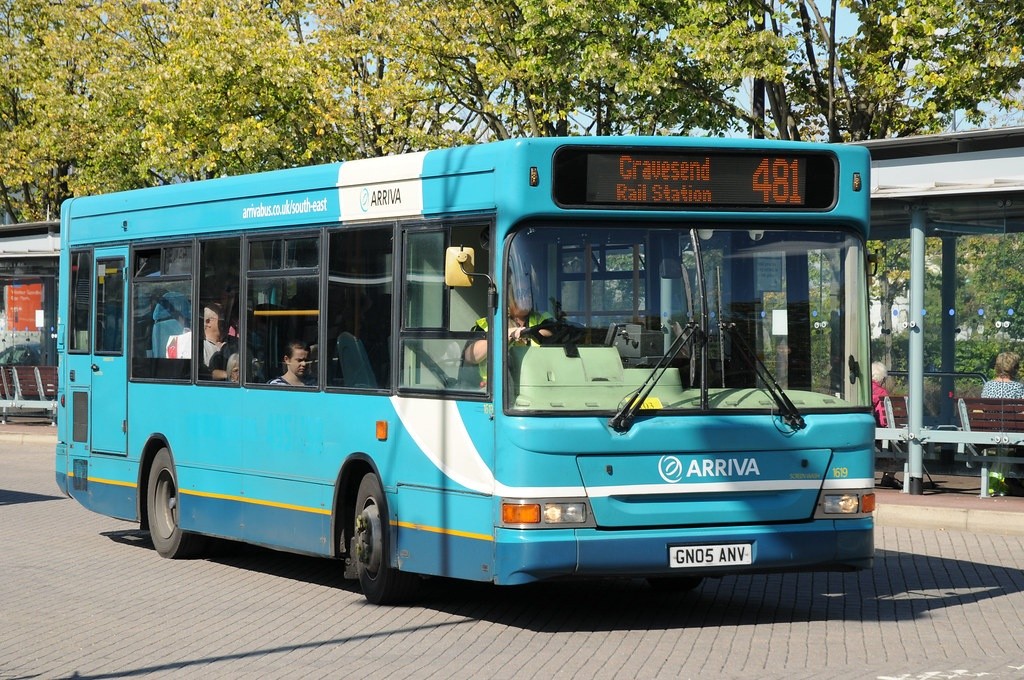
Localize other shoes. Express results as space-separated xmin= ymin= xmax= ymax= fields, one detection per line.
xmin=881 ymin=473 xmax=904 ymax=490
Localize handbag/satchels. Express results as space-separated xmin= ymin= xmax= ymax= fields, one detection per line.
xmin=988 ymin=451 xmax=1011 ymax=497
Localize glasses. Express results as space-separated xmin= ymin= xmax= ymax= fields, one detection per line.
xmin=513 ymin=288 xmax=537 ymax=298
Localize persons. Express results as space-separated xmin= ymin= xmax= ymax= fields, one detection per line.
xmin=183 ymin=277 xmax=318 ymax=384
xmin=267 ymin=340 xmax=329 ymax=386
xmin=972 ymin=352 xmax=1024 ymax=497
xmin=461 ymin=276 xmax=556 ymax=406
xmin=871 ymin=360 xmax=902 ymax=488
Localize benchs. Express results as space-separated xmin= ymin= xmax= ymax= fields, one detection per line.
xmin=0 ymin=364 xmax=58 ymax=426
xmin=958 ymin=397 xmax=1024 ymax=497
xmin=871 ymin=395 xmax=910 ymax=493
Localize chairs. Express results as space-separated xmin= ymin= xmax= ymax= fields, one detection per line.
xmin=152 ymin=291 xmax=191 ymax=359
xmin=337 ymin=332 xmax=382 ymax=388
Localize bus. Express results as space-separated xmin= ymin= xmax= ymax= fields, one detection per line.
xmin=54 ymin=136 xmax=879 ymax=606
